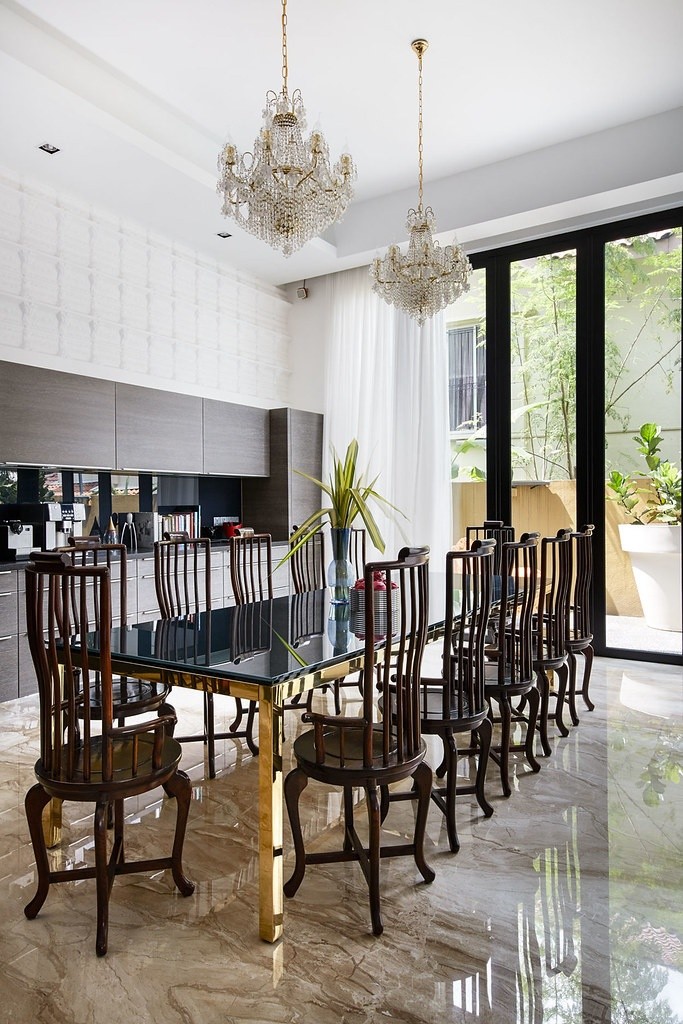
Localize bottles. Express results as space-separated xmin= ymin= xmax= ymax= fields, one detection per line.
xmin=103 ymin=515 xmax=119 ymax=557
xmin=89 ymin=516 xmax=102 ymax=555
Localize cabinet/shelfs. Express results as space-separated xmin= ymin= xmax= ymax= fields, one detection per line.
xmin=0 ymin=360 xmax=325 ymax=703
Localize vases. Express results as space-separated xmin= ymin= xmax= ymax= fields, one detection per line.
xmin=325 ymin=528 xmax=356 ymax=602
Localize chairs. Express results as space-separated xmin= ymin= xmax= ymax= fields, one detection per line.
xmin=532 ymin=522 xmax=595 ymax=726
xmin=452 ymin=521 xmax=516 ymax=662
xmin=378 ymin=538 xmax=496 ymax=853
xmin=284 ymin=546 xmax=435 ymax=936
xmin=290 ymin=525 xmax=365 ymax=714
xmin=442 ymin=536 xmax=551 ymax=776
xmin=22 ymin=550 xmax=194 ymax=958
xmin=229 ymin=527 xmax=315 ymax=743
xmin=52 ymin=536 xmax=171 ymax=829
xmin=153 ymin=536 xmax=259 ymax=779
xmin=503 ymin=526 xmax=579 ymax=736
xmin=330 ymin=527 xmax=399 ymax=689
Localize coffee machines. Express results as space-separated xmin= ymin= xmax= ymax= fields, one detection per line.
xmin=18 ymin=501 xmax=86 ymax=552
xmin=0 ymin=520 xmax=42 ymax=561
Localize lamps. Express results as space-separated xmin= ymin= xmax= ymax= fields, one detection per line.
xmin=215 ymin=0 xmax=359 ymax=260
xmin=369 ymin=39 xmax=473 ymax=327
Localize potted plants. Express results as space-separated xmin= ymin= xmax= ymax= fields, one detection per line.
xmin=606 ymin=422 xmax=682 ymax=635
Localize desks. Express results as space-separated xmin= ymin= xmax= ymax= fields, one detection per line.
xmin=39 ymin=573 xmax=558 ymax=941
